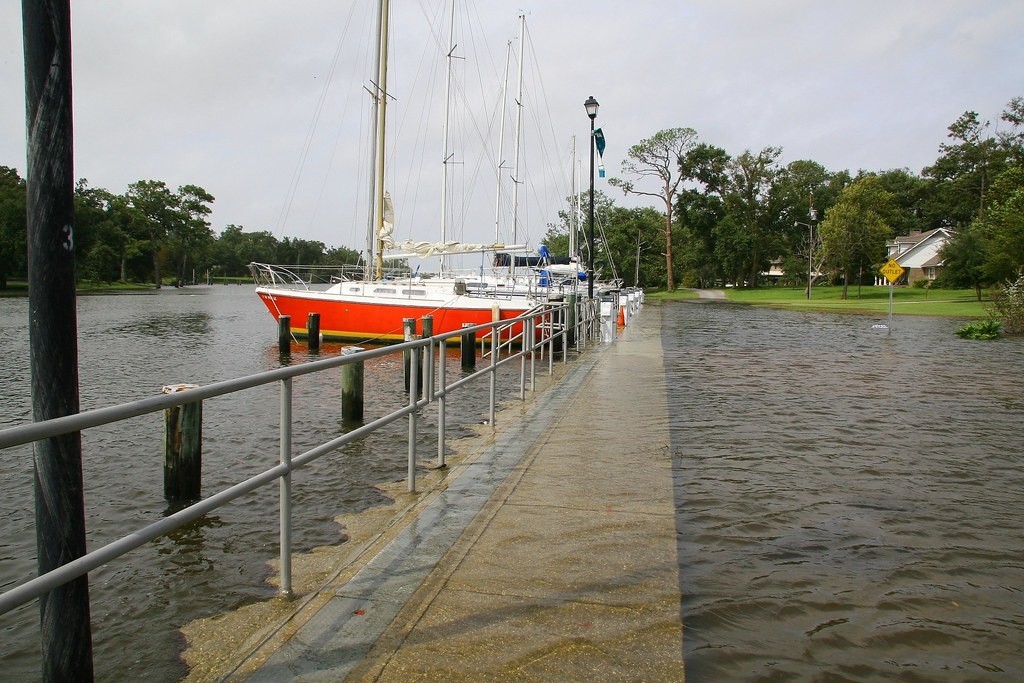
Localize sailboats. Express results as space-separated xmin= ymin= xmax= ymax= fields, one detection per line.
xmin=246 ymin=0 xmax=627 ymax=348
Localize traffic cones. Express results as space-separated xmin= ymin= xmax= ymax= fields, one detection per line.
xmin=616 ymin=305 xmax=627 ymax=330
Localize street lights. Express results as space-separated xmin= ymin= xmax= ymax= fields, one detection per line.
xmin=794 ymin=220 xmax=812 ymax=300
xmin=582 ymin=94 xmax=601 ymax=336
